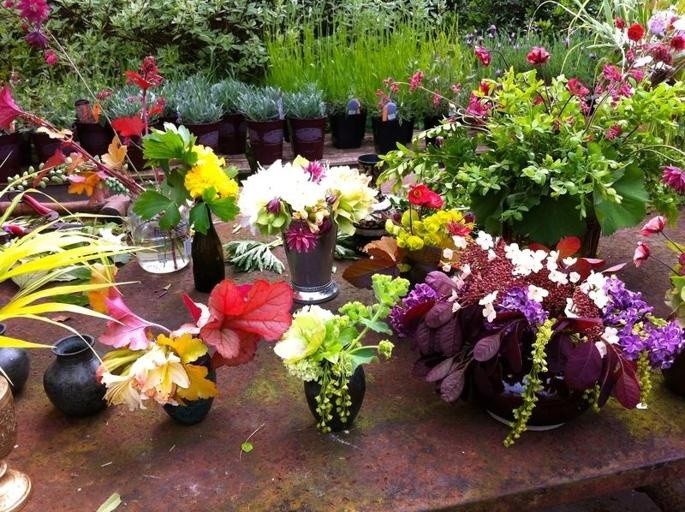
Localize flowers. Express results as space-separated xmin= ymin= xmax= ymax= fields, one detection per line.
xmin=0 ymin=0 xmax=685 ymax=449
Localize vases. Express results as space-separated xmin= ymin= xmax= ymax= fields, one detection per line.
xmin=0 ymin=375 xmax=33 ymax=512
